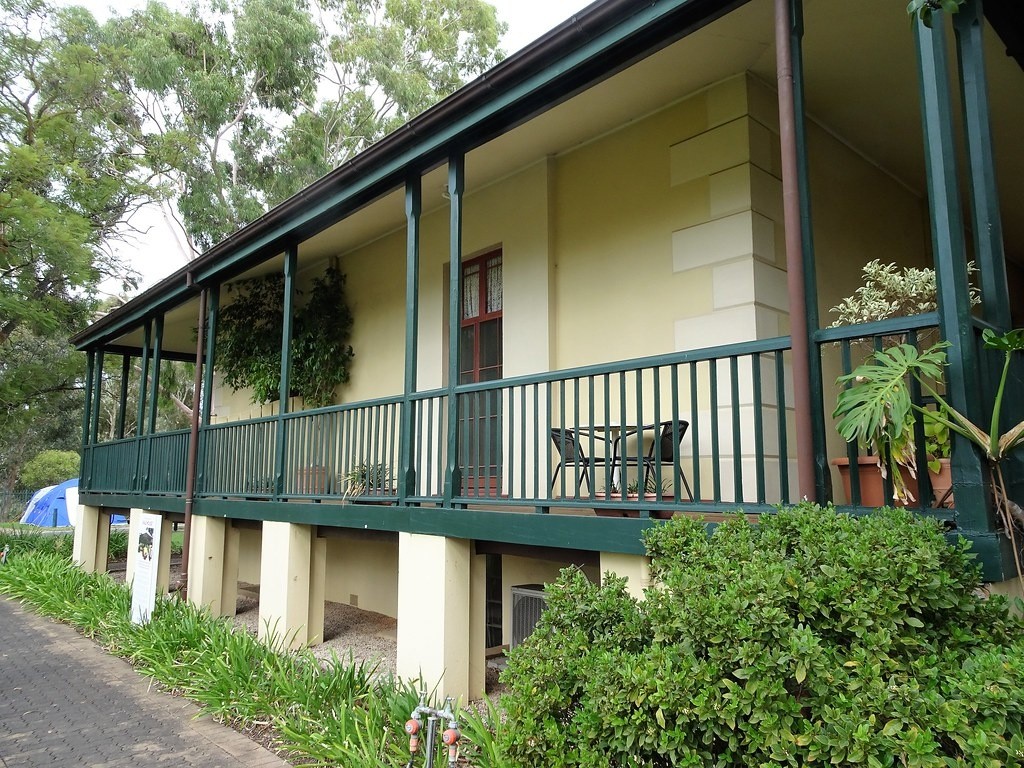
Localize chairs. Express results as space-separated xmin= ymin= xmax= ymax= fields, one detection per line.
xmin=610 ymin=420 xmax=698 ymax=504
xmin=549 ymin=426 xmax=614 ymax=497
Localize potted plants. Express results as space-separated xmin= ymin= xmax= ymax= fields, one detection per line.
xmin=590 ymin=474 xmax=674 ymax=517
xmin=334 ymin=455 xmax=399 ymax=508
xmin=246 ymin=478 xmax=274 ymax=501
xmin=819 ymin=259 xmax=982 ymax=510
xmin=281 ymin=328 xmax=356 ymax=497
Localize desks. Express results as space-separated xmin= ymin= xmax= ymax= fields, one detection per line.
xmin=571 ymin=425 xmax=660 ymax=494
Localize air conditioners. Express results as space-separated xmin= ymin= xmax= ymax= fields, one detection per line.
xmin=508 ymin=582 xmax=557 ymax=658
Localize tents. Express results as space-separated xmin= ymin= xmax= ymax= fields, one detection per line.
xmin=20 ymin=478 xmax=128 ymax=527
